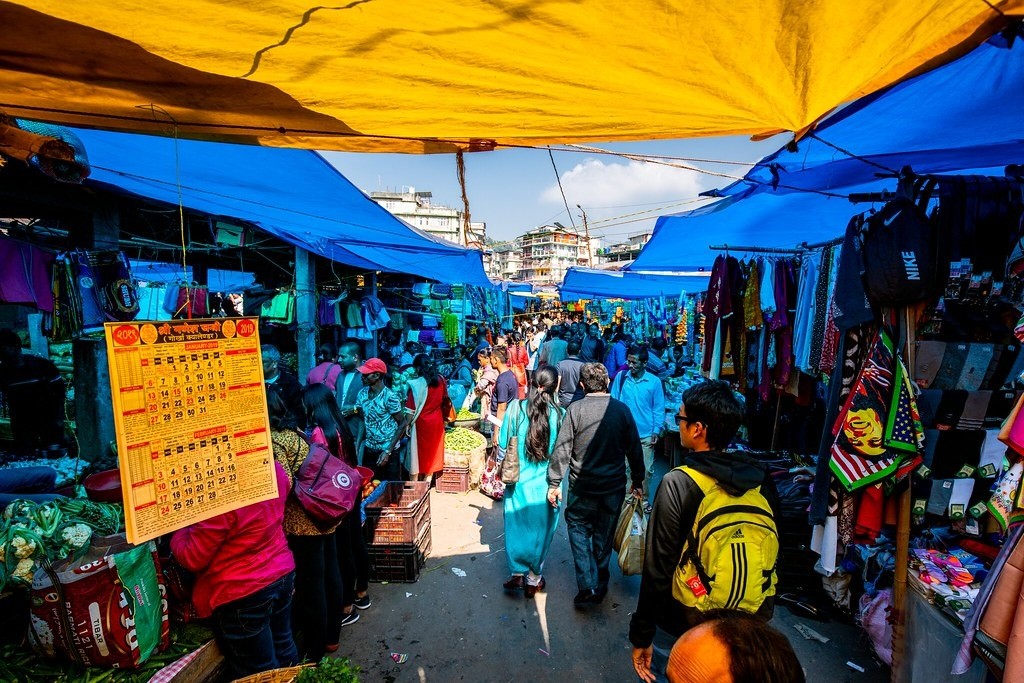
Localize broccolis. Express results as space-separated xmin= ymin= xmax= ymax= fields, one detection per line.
xmin=0 ymin=499 xmax=93 ymax=586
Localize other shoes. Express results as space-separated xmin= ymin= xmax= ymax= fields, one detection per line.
xmin=326 ymin=643 xmax=339 ymax=652
xmin=525 ymin=577 xmax=545 ymax=598
xmin=574 ymin=588 xmax=602 ymax=608
xmin=503 ymin=575 xmax=526 ymax=590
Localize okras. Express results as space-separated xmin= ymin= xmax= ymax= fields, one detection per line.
xmin=0 ymin=623 xmax=214 ymax=683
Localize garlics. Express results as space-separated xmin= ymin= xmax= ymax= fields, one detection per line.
xmin=0 ymin=456 xmax=91 ymax=484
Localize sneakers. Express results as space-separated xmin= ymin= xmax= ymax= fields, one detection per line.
xmin=341 ymin=607 xmax=359 ymax=627
xmin=352 ymin=595 xmax=371 ymax=610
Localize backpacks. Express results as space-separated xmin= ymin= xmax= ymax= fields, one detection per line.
xmin=669 ymin=464 xmax=779 ymax=616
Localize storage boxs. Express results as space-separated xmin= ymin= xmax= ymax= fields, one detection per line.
xmin=436 ymin=428 xmax=485 ymax=492
xmin=363 ymin=480 xmax=433 ymax=586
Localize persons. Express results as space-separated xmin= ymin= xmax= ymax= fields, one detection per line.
xmin=260 ymin=343 xmax=371 ymax=659
xmin=0 ymin=327 xmax=66 ymax=445
xmin=664 ymin=605 xmax=807 ymax=682
xmin=545 ymin=362 xmax=646 ymax=611
xmin=498 ymin=365 xmax=566 ymax=598
xmin=610 ymin=344 xmax=666 ymax=513
xmin=310 ymin=336 xmax=476 ymax=481
xmin=170 ymin=462 xmax=298 ymax=678
xmin=556 ymin=314 xmax=691 ymax=410
xmin=626 ymin=379 xmax=789 ymax=683
xmin=467 ymin=312 xmax=568 ymax=451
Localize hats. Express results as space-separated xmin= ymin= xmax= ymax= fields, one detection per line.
xmin=355 ymin=358 xmax=388 ymax=375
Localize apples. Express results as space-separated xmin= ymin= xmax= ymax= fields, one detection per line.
xmin=361 ymin=480 xmax=403 ymax=541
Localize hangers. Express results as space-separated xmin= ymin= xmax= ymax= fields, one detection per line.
xmin=722 ymin=244 xmax=799 ymax=263
xmin=863 ymin=188 xmax=895 ymax=216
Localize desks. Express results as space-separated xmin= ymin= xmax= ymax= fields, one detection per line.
xmin=851 ymin=547 xmax=1006 ymax=683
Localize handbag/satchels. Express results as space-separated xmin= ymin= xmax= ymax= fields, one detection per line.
xmin=438 ymin=374 xmax=457 ymax=423
xmin=612 ymin=491 xmax=648 ymax=576
xmin=291 ymin=444 xmax=363 ymax=532
xmin=498 ymin=436 xmax=518 ymax=485
xmin=479 ymin=446 xmax=506 ymax=499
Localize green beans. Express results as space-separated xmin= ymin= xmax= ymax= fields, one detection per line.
xmin=444 ymin=425 xmax=484 ymax=453
xmin=457 ymin=408 xmax=478 ymax=420
xmin=61 ymin=499 xmax=122 ymax=535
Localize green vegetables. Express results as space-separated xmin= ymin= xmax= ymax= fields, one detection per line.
xmin=294 ymin=656 xmax=360 ymax=683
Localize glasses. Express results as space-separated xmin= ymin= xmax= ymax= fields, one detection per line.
xmin=675 ymin=413 xmax=708 ymax=428
